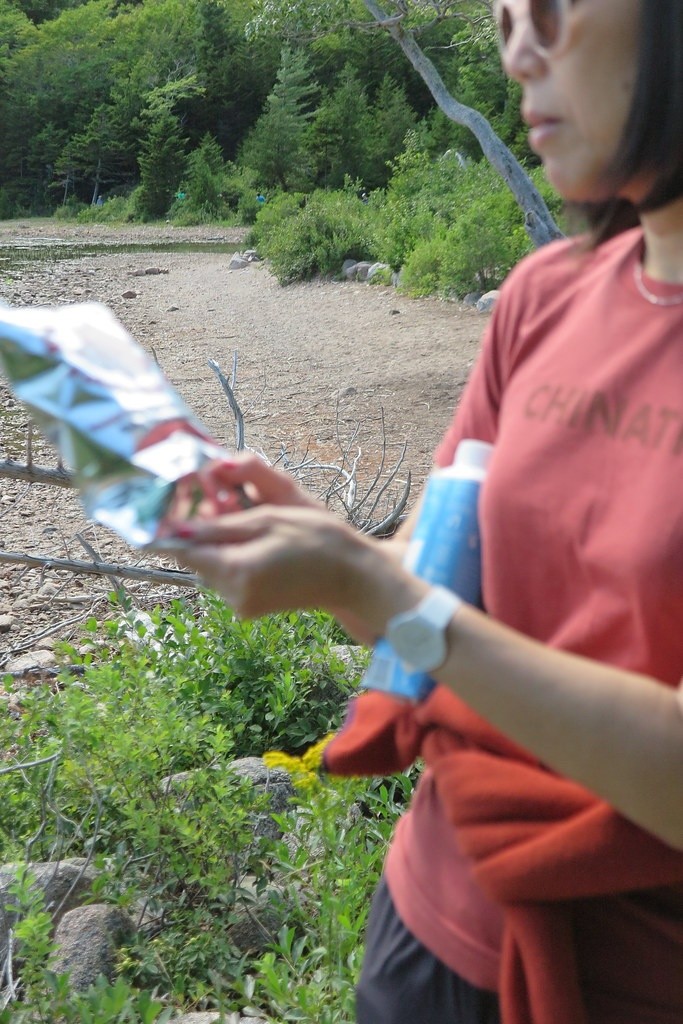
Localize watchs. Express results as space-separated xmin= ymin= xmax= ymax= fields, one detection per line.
xmin=388 ymin=585 xmax=461 ymax=673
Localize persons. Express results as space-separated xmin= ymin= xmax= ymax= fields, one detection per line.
xmin=162 ymin=0 xmax=683 ymax=1024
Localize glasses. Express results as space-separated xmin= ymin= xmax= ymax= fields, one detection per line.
xmin=492 ymin=0 xmax=572 ymax=59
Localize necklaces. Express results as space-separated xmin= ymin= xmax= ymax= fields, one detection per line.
xmin=634 ymin=264 xmax=682 ymax=306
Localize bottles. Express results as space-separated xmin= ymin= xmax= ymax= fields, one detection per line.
xmin=356 ymin=438 xmax=493 ymax=703
